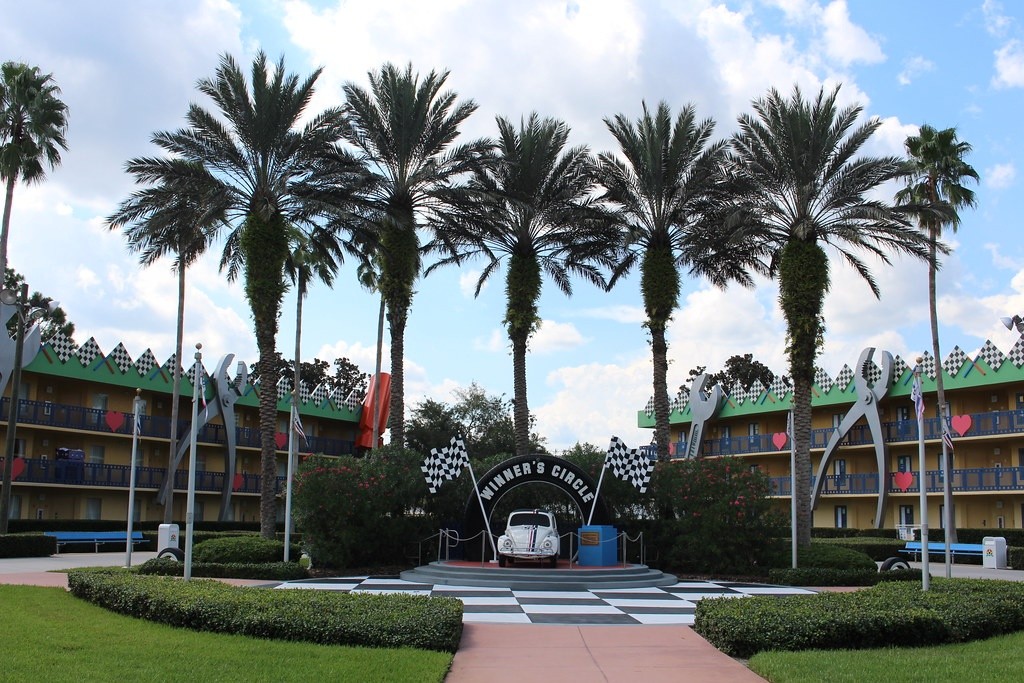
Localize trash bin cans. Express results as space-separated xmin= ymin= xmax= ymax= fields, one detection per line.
xmin=157 ymin=523 xmax=180 ymax=556
xmin=982 ymin=536 xmax=1007 ymax=569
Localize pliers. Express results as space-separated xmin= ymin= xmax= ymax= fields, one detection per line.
xmin=810 ymin=346 xmax=896 ymax=530
xmin=155 ymin=352 xmax=248 ymax=521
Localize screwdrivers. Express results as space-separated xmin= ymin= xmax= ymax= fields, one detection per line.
xmin=355 ymin=372 xmax=392 ymax=459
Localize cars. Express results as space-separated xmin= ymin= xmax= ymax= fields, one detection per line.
xmin=497 ymin=508 xmax=560 ymax=568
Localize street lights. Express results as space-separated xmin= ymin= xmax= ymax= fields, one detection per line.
xmin=0 ymin=284 xmax=46 ymax=534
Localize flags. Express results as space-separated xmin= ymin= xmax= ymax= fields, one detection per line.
xmin=942 ymin=415 xmax=953 ymax=456
xmin=419 ymin=429 xmax=470 ymax=493
xmin=293 ymin=407 xmax=309 ymax=447
xmin=135 ymin=407 xmax=142 ymax=444
xmin=605 ymin=436 xmax=655 ymax=494
xmin=197 ymin=366 xmax=208 ymax=424
xmin=910 ymin=373 xmax=926 ymax=423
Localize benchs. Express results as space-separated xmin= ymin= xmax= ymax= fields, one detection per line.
xmin=898 ymin=541 xmax=983 ymax=565
xmin=44 ymin=531 xmax=151 ymax=554
